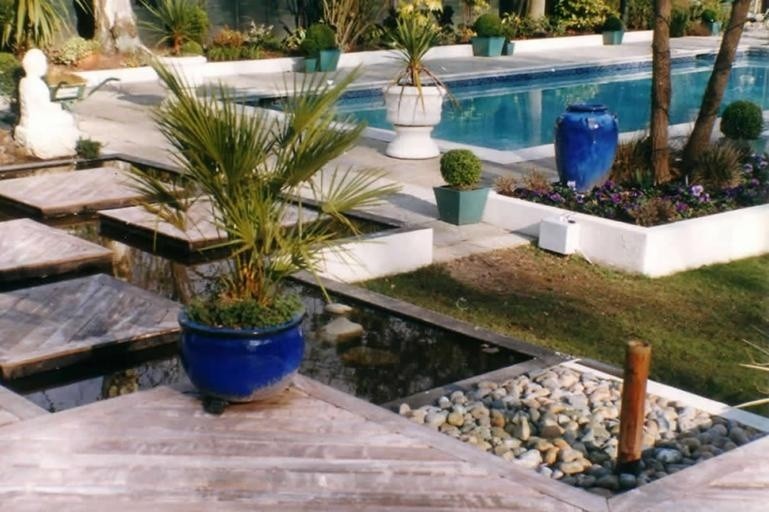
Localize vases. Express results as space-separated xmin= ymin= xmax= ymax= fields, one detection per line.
xmin=554 ymin=104 xmax=620 ymax=200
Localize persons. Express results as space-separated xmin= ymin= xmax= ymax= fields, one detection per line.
xmin=11 ymin=47 xmax=80 ymax=161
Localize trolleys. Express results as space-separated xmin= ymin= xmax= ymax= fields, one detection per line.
xmin=43 ymin=70 xmax=122 ymax=114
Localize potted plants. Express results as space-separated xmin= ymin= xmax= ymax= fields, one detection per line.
xmin=702 ymin=8 xmax=720 ymax=36
xmin=61 ymin=37 xmax=100 ymax=72
xmin=719 ymin=101 xmax=768 ymax=156
xmin=602 ymin=14 xmax=625 ymax=45
